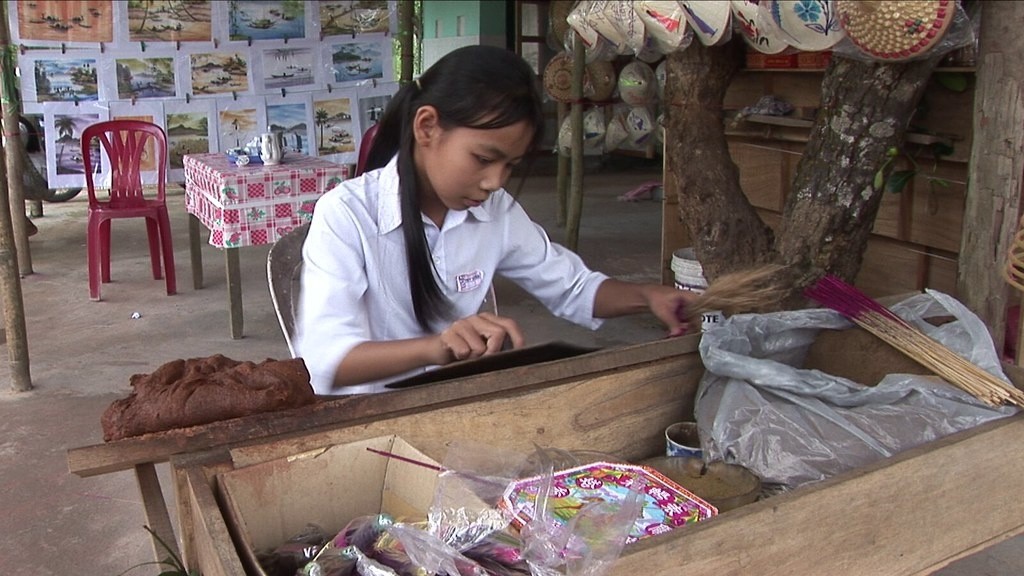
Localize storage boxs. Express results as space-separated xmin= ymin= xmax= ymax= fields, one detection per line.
xmin=746 ymin=50 xmax=832 ymax=68
xmin=171 ymin=327 xmax=1024 ymax=576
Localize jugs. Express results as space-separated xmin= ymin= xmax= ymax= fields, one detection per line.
xmin=259 ymin=126 xmax=282 ymax=166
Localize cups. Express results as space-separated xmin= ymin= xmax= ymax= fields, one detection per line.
xmin=658 ymin=421 xmax=703 ymax=461
xmin=235 ymin=155 xmax=250 ymax=167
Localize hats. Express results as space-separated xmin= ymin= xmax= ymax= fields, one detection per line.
xmin=543 ymin=53 xmax=587 ymax=100
xmin=568 ymin=0 xmax=957 ymax=63
xmin=585 ymin=60 xmax=667 ymax=106
xmin=558 ymin=106 xmax=665 ymax=158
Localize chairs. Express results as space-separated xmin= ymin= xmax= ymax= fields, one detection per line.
xmin=81 ymin=120 xmax=176 ymax=301
xmin=267 ymin=223 xmax=498 ymax=360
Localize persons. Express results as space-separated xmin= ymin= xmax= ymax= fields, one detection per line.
xmin=292 ymin=45 xmax=700 ymax=395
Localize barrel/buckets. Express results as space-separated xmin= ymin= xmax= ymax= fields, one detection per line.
xmin=638 ymin=457 xmax=763 ymax=515
xmin=671 ymin=248 xmax=727 ymax=333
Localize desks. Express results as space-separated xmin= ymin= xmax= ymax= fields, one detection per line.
xmin=183 ymin=152 xmax=348 ymax=339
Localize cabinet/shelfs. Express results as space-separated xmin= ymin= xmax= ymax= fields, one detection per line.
xmin=724 ymin=68 xmax=978 ymax=147
